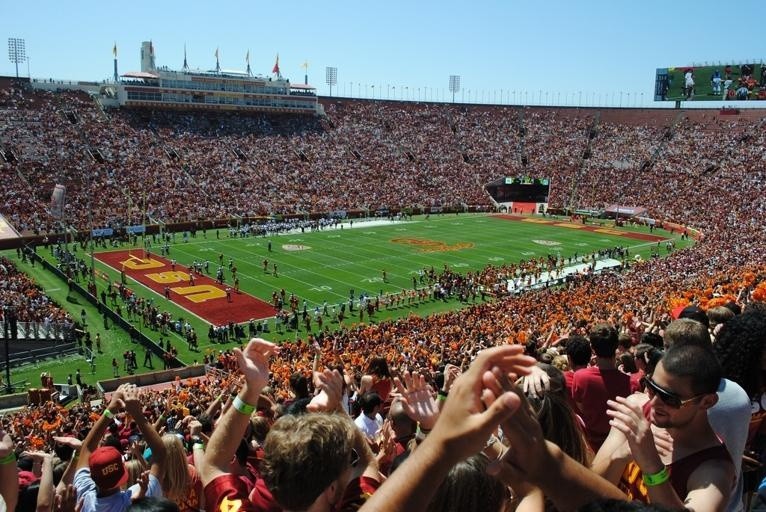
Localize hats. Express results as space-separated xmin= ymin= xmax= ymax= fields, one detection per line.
xmin=88 ymin=447 xmax=129 ymax=490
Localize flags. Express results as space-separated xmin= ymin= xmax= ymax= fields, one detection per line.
xmin=112 ymin=44 xmax=117 ymax=56
xmin=272 ymin=55 xmax=279 ymax=73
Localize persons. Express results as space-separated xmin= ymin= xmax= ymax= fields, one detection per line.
xmin=681 ymin=64 xmax=766 ymax=100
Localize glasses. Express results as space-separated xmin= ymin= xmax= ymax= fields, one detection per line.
xmin=644 ymin=376 xmax=701 ymax=409
